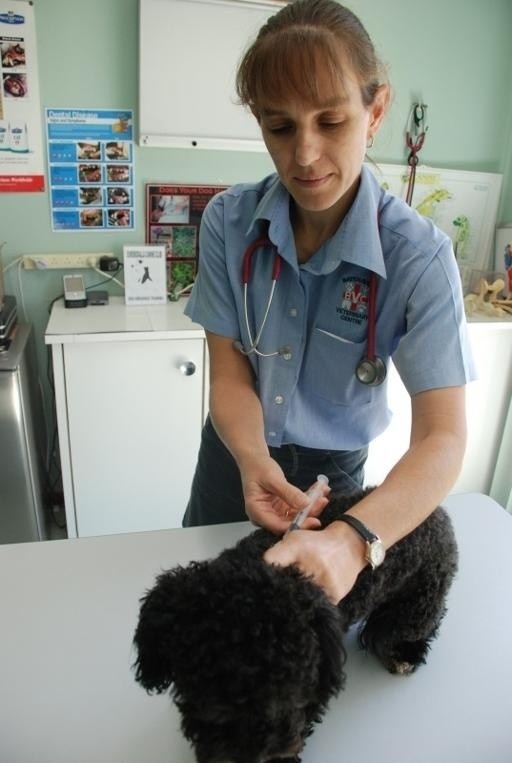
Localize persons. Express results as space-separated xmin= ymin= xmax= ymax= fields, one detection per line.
xmin=177 ymin=0 xmax=474 ymax=617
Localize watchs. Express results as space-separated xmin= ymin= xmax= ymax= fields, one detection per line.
xmin=329 ymin=512 xmax=389 ymax=571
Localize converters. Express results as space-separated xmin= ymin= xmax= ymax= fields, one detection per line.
xmin=100 ymin=257 xmax=119 ymax=271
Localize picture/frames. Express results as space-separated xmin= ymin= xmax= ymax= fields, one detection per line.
xmin=362 ymin=160 xmax=506 ymax=278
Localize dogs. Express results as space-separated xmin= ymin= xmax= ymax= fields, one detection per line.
xmin=453 ymin=214 xmax=471 ymax=260
xmin=130 ymin=485 xmax=459 ymax=763
xmin=415 ymin=189 xmax=454 ymax=218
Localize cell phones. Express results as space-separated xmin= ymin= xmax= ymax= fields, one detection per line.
xmin=63 ymin=274 xmax=88 ymax=308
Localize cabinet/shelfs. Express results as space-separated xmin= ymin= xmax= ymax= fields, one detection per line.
xmin=45 ymin=293 xmax=211 ymax=536
xmin=359 ymin=317 xmax=511 ymax=508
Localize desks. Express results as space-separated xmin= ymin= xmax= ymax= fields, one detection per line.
xmin=1 ymin=489 xmax=510 ymax=763
